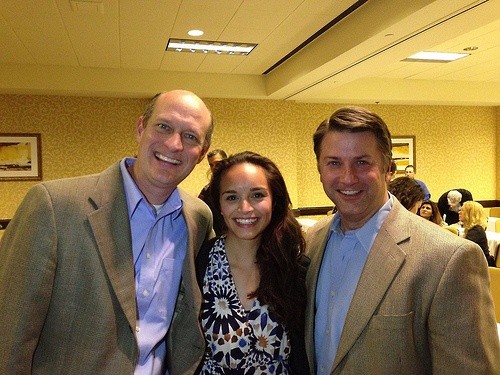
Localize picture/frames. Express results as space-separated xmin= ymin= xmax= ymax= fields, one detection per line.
xmin=391 ymin=135 xmax=416 ymax=174
xmin=0 ymin=133 xmax=42 ymax=182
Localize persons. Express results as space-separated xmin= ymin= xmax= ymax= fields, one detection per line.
xmin=437 ymin=189 xmax=474 ymax=225
xmin=457 ymin=201 xmax=497 ymax=268
xmin=0 ymin=89 xmax=215 ymax=375
xmin=196 ymin=151 xmax=312 ymax=375
xmin=302 ymin=106 xmax=500 ymax=375
xmin=404 ymin=165 xmax=431 ymax=201
xmin=391 ymin=162 xmax=398 ymax=181
xmin=388 ymin=177 xmax=426 ymax=216
xmin=416 ymin=199 xmax=442 ymax=226
xmin=197 ymin=149 xmax=228 ymax=200
